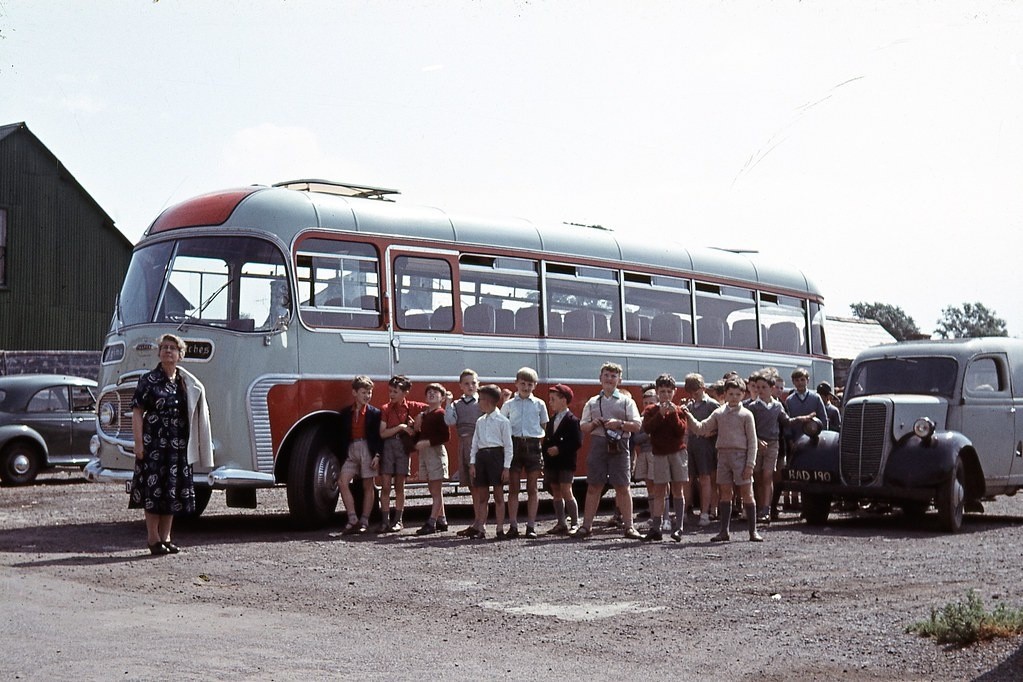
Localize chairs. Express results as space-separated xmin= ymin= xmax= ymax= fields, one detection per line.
xmin=321 ymin=295 xmax=828 ymax=357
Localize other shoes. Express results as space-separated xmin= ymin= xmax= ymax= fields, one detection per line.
xmin=637 ymin=511 xmax=650 ymax=518
xmin=829 ymin=503 xmax=845 ymax=514
xmin=671 ymin=529 xmax=683 ymax=542
xmin=416 ymin=523 xmax=436 ymax=535
xmin=376 ymin=523 xmax=390 ymax=533
xmin=698 ymin=500 xmax=801 ymax=526
xmin=470 ymin=523 xmax=579 ymax=539
xmin=624 ymin=529 xmax=641 ymax=538
xmin=750 ymin=532 xmax=763 ymax=542
xmin=641 ymin=528 xmax=662 ymax=542
xmin=343 ymin=521 xmax=357 ymax=534
xmin=436 ymin=521 xmax=449 ymax=531
xmin=390 ymin=522 xmax=404 ymax=531
xmin=163 ymin=541 xmax=180 ymax=553
xmin=609 ymin=514 xmax=625 ymax=527
xmin=663 ymin=520 xmax=671 ymax=531
xmin=358 ymin=523 xmax=369 ymax=533
xmin=710 ymin=534 xmax=730 ymax=542
xmin=571 ymin=526 xmax=593 ymax=538
xmin=457 ymin=526 xmax=474 ymax=537
xmin=148 ymin=541 xmax=170 ymax=555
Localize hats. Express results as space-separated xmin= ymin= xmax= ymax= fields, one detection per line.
xmin=816 ymin=383 xmax=831 ymax=394
xmin=549 ymin=384 xmax=573 ymax=404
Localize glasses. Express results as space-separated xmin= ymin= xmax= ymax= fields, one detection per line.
xmin=160 ymin=345 xmax=179 ymax=350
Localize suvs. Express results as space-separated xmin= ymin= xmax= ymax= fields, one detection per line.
xmin=780 ymin=336 xmax=1023 ymax=534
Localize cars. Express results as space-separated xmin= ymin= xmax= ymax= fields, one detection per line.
xmin=1 ymin=375 xmax=98 ymax=486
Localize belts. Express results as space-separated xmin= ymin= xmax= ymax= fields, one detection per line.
xmin=353 ymin=438 xmax=366 ymax=442
xmin=390 ymin=433 xmax=400 ymax=439
xmin=511 ymin=437 xmax=539 ymax=442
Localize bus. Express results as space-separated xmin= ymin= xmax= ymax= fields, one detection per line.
xmin=81 ymin=180 xmax=835 ymax=530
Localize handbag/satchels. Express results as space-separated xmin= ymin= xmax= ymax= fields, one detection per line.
xmin=399 ymin=402 xmax=421 ymax=455
xmin=607 ymin=441 xmax=623 ymax=455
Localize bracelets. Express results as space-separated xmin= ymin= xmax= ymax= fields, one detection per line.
xmin=376 ymin=455 xmax=380 ymax=457
xmin=420 ymin=412 xmax=425 ymax=416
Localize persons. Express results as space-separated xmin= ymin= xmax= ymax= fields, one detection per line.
xmin=338 ymin=375 xmax=385 ymax=536
xmin=500 ymin=367 xmax=550 ymax=539
xmin=541 ymin=384 xmax=579 ymax=536
xmin=378 ymin=375 xmax=428 ymax=532
xmin=636 ymin=389 xmax=672 ymax=530
xmin=128 ymin=334 xmax=196 ymax=553
xmin=641 ymin=374 xmax=688 ymax=541
xmin=679 ymin=373 xmax=720 ymax=526
xmin=680 ymin=375 xmax=763 ymax=542
xmin=568 ymin=362 xmax=644 ymax=538
xmin=705 ymin=367 xmax=841 ymax=524
xmin=415 ymin=383 xmax=450 ymax=534
xmin=444 ymin=369 xmax=486 ymax=536
xmin=611 ymin=388 xmax=635 ymax=526
xmin=456 ymin=385 xmax=513 ymax=540
